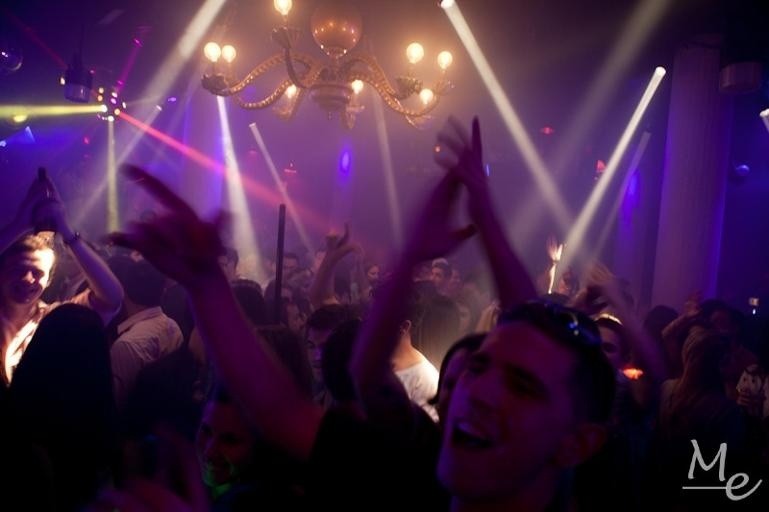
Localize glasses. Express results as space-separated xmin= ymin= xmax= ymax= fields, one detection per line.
xmin=526 ymin=297 xmax=602 ymax=347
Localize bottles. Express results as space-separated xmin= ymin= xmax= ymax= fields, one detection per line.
xmin=30 ymin=164 xmax=58 ymax=236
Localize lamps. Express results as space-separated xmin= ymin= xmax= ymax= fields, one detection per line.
xmin=59 ymin=67 xmax=94 ymax=106
xmin=197 ymin=0 xmax=458 ymax=129
xmin=92 ymin=84 xmax=128 ymax=124
xmin=0 ymin=43 xmax=27 ymax=73
